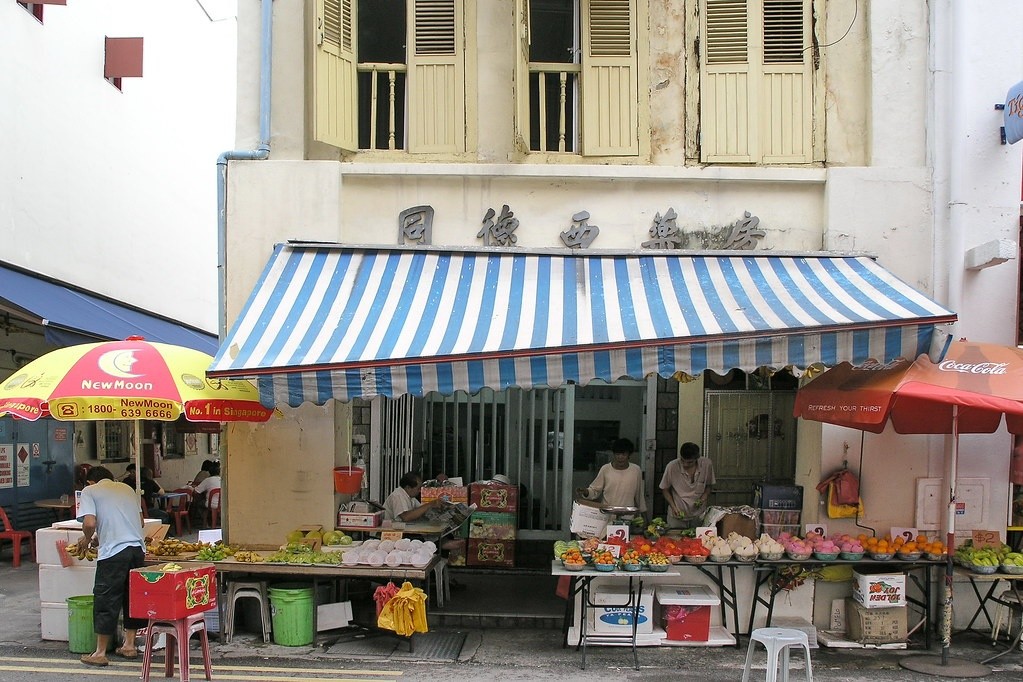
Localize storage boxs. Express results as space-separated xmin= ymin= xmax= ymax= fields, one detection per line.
xmin=751 ymin=480 xmax=805 ymax=510
xmin=662 ymin=604 xmax=711 ymax=641
xmin=41 ymin=603 xmax=73 ymax=643
xmin=593 ymin=584 xmax=655 ymax=635
xmin=420 ymin=485 xmax=519 ymax=570
xmin=570 ymin=498 xmax=610 ymax=538
xmin=852 ymin=568 xmax=906 ymax=609
xmin=35 ymin=520 xmax=162 ymax=563
xmin=761 ymin=524 xmax=802 ymax=540
xmin=37 ymin=565 xmax=95 ymax=602
xmin=712 ymin=505 xmax=759 ymax=540
xmin=339 ymin=509 xmax=385 ymax=528
xmin=844 ymin=597 xmax=908 ymax=643
xmin=129 ymin=561 xmax=216 ymax=620
xmin=759 ymin=508 xmax=799 ymax=525
xmin=763 ymin=616 xmax=819 ymax=648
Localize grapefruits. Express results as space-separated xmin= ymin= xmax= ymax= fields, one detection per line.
xmin=286 ymin=529 xmax=352 ymax=545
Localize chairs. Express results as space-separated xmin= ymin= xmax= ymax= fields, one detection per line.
xmin=203 ymin=488 xmax=221 ymax=529
xmin=167 ymin=488 xmax=192 ymax=538
xmin=0 ymin=507 xmax=35 ymax=568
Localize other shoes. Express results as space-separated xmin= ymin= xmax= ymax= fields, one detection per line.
xmin=115 ymin=645 xmax=139 ymax=659
xmin=448 ymin=578 xmax=467 ymax=589
xmin=81 ymin=650 xmax=108 ymax=667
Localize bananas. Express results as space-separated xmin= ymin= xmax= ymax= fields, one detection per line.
xmin=145 ymin=537 xmax=265 ymax=564
xmin=64 ymin=543 xmax=98 ymax=562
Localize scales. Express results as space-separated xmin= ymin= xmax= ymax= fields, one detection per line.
xmin=600 ymin=506 xmax=639 ymax=543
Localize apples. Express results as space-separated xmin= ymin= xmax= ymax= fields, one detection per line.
xmin=779 ymin=532 xmax=863 ymax=553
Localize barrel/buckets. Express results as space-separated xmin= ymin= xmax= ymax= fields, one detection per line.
xmin=65 ymin=595 xmax=113 ymax=654
xmin=268 ymin=582 xmax=313 ymax=646
xmin=334 ymin=452 xmax=365 ymax=494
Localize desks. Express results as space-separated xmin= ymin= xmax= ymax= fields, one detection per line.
xmin=564 ymin=560 xmax=753 ymax=650
xmin=152 ymin=492 xmax=187 ymax=508
xmin=749 ymin=558 xmax=948 ymax=650
xmin=953 ymin=568 xmax=1023 ymax=643
xmin=336 ymin=523 xmax=451 ymax=560
xmin=551 ymin=560 xmax=680 ymax=671
xmin=138 ymin=548 xmax=435 ymax=652
xmin=35 ymin=498 xmax=75 ymax=521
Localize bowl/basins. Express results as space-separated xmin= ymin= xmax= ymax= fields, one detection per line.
xmin=561 ymin=551 xmax=947 ymax=569
xmin=952 ymin=558 xmax=1023 ymax=574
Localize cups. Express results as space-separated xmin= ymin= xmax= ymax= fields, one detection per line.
xmin=60 ymin=495 xmax=69 ymax=503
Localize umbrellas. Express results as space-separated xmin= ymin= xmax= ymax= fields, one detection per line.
xmin=790 ymin=336 xmax=1023 ymax=665
xmin=0 ymin=334 xmax=285 ymax=503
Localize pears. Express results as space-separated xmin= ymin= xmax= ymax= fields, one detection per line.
xmin=703 ymin=531 xmax=786 ymax=556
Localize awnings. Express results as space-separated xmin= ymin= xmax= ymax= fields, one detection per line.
xmin=1 ymin=266 xmax=219 ymax=359
xmin=202 ymin=235 xmax=955 ymax=409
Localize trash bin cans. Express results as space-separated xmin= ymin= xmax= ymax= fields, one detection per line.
xmin=65 ymin=594 xmax=119 ymax=653
xmin=266 ymin=582 xmax=314 ymax=646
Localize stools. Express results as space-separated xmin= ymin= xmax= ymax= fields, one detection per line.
xmin=224 ymin=580 xmax=272 ymax=645
xmin=430 ymin=562 xmax=451 ymax=608
xmin=743 ymin=629 xmax=812 ymax=681
xmin=991 ymin=590 xmax=1023 ymax=648
xmin=142 ymin=613 xmax=212 ymax=681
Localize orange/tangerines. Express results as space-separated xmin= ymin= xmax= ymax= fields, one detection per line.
xmin=561 ymin=549 xmax=641 ymax=566
xmin=856 ymin=534 xmax=948 ymax=554
xmin=666 ymin=543 xmax=675 ymax=548
xmin=641 ymin=544 xmax=650 ymax=551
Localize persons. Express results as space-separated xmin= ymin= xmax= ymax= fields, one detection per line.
xmin=75 ymin=466 xmax=145 ymax=668
xmin=190 ymin=458 xmax=221 ymax=530
xmin=380 ymin=470 xmax=444 ymax=522
xmin=656 ymin=441 xmax=717 ymax=528
xmin=121 ymin=463 xmax=170 ymax=524
xmin=575 ymin=435 xmax=649 ymax=524
